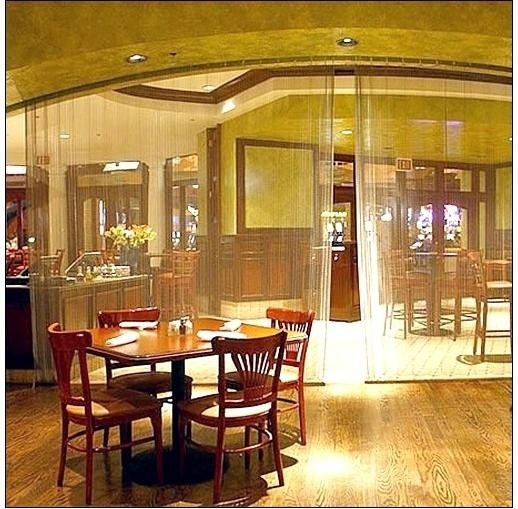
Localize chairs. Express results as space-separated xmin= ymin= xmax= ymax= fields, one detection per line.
xmin=384 ymin=248 xmax=513 ymax=362
xmin=47 ymin=322 xmax=165 ymax=503
xmin=96 ymin=307 xmax=194 ymax=448
xmin=153 ymin=250 xmax=200 ymax=319
xmin=181 ymin=330 xmax=289 ymax=503
xmin=257 ymin=305 xmax=315 ymax=464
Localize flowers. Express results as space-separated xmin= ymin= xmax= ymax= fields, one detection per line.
xmin=104 ymin=223 xmax=158 ymax=252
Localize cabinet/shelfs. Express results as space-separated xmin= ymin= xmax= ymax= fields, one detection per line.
xmin=6 ymin=280 xmax=147 ymax=387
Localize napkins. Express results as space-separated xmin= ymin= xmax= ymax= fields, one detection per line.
xmin=105 ymin=330 xmax=139 ymax=347
xmin=118 ymin=321 xmax=159 ymax=331
xmin=219 ymin=319 xmax=244 ymax=332
xmin=197 ymin=330 xmax=247 ymax=341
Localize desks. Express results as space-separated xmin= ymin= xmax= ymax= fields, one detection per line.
xmin=77 ymin=315 xmax=310 ymax=493
